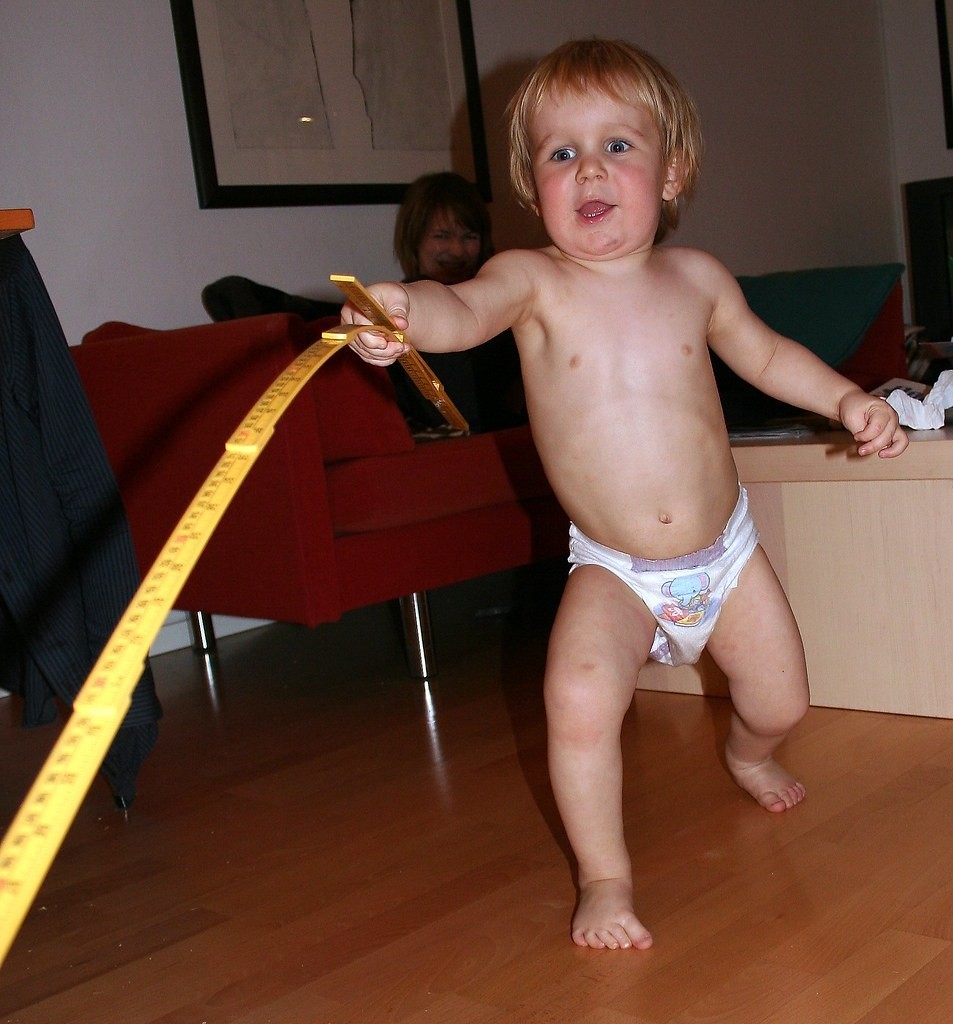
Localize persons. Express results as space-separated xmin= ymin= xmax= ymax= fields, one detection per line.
xmin=345 ymin=35 xmax=909 ymax=950
xmin=384 ymin=173 xmax=572 ymax=650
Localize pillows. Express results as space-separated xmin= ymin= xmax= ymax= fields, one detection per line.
xmin=286 ymin=311 xmax=415 ymax=463
xmin=713 ymin=260 xmax=904 ymax=399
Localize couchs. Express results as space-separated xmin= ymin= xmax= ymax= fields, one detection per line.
xmin=66 ymin=276 xmax=909 ymax=678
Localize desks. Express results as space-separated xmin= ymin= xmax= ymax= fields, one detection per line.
xmin=635 ymin=423 xmax=953 ymax=723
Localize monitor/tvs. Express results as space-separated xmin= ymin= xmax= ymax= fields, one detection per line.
xmin=904 ymin=176 xmax=953 ymax=343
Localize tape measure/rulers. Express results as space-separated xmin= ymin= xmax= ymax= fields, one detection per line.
xmin=0 ymin=274 xmax=470 ymax=973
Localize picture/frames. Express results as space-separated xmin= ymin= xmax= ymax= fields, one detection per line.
xmin=170 ymin=0 xmax=493 ymax=210
